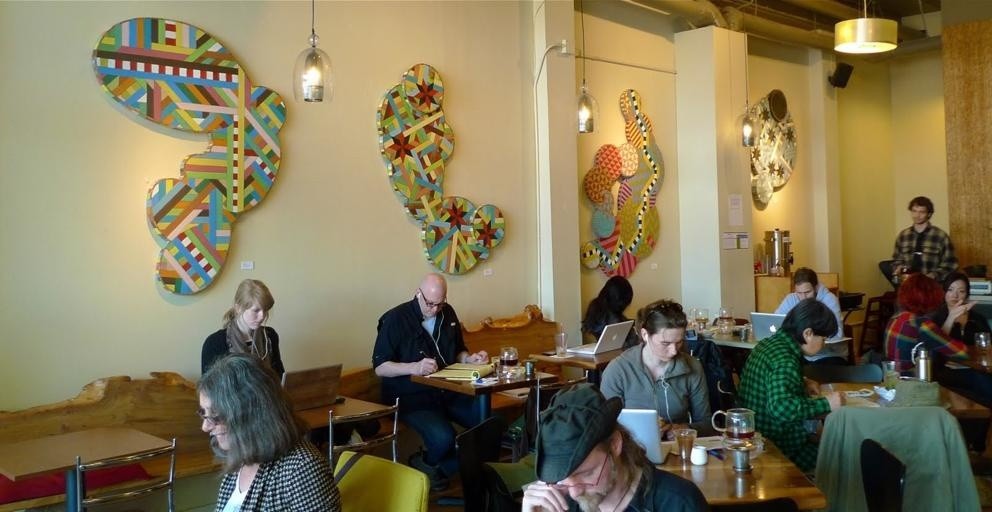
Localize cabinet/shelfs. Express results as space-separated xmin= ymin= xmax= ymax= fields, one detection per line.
xmin=756 ymin=273 xmax=839 ymax=313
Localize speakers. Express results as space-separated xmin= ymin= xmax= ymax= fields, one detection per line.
xmin=830 ymin=63 xmax=854 ymax=90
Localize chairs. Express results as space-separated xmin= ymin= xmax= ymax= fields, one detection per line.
xmin=328 ymin=398 xmax=399 ymax=463
xmin=861 ymin=438 xmax=907 ymax=512
xmin=75 ymin=437 xmax=177 ymax=512
xmin=332 ymin=452 xmax=430 ymax=512
xmin=531 ymin=372 xmax=588 ymax=430
xmin=715 ymin=379 xmax=739 ymax=434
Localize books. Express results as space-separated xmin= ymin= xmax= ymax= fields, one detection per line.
xmin=429 ymin=362 xmax=496 ymax=379
xmin=663 ymin=436 xmax=724 ymax=454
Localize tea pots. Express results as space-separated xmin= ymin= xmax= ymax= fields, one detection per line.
xmin=689 ymin=308 xmax=709 ymax=334
xmin=711 ymin=409 xmax=763 ymax=460
xmin=498 ymin=348 xmax=521 ymax=380
xmin=717 ymin=306 xmax=735 ymax=334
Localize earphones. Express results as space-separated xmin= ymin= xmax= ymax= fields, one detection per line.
xmin=643 ymin=332 xmax=683 ymax=427
xmin=235 ymin=307 xmax=269 ymax=364
xmin=415 ymin=293 xmax=453 ymax=368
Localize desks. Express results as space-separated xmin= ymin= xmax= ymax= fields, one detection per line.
xmin=296 ymin=395 xmax=389 ymax=430
xmin=685 ymin=321 xmax=852 ymax=371
xmin=819 ymin=376 xmax=989 ymax=421
xmin=657 ymin=432 xmax=826 ymax=511
xmin=412 ymin=362 xmax=559 ymax=418
xmin=0 ymin=426 xmax=172 ymax=511
xmin=529 ymin=347 xmax=624 ymax=370
xmin=961 ymin=343 xmax=991 ymax=374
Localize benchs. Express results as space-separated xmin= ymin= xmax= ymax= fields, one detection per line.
xmin=1 ymin=306 xmax=558 ymax=512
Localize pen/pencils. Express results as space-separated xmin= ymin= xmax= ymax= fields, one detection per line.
xmin=420 ymin=350 xmax=440 ymax=372
xmin=708 ymin=450 xmax=725 ymax=460
xmin=828 ymin=383 xmax=834 ymax=393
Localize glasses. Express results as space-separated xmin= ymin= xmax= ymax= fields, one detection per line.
xmin=196 ymin=408 xmax=222 ymax=425
xmin=553 ymin=453 xmax=609 ymax=489
xmin=419 ymin=287 xmax=447 ymax=307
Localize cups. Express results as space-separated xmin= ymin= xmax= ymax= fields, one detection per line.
xmin=674 ymin=429 xmax=694 ymax=461
xmin=555 ymin=332 xmax=568 ymax=357
xmin=880 ymin=360 xmax=902 ymax=391
xmin=690 ymin=444 xmax=707 ymax=466
xmin=975 ymin=331 xmax=990 ymax=365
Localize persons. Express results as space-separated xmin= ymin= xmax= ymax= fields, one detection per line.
xmin=196 ymin=352 xmax=342 ymax=512
xmin=931 ymin=271 xmax=992 ymax=346
xmin=372 ymin=273 xmax=505 ymax=492
xmin=892 ymin=197 xmax=957 ymax=283
xmin=735 ymin=298 xmax=847 ymax=483
xmin=882 ymin=272 xmax=970 ymax=376
xmin=521 ymin=381 xmax=710 ymax=512
xmin=774 ymin=267 xmax=849 ymax=362
xmin=600 ymin=300 xmax=711 ymax=437
xmin=202 ymin=278 xmax=286 ymax=461
xmin=581 ymin=275 xmax=639 ymax=344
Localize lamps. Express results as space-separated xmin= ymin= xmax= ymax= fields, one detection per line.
xmin=575 ymin=92 xmax=599 ymax=135
xmin=290 ymin=34 xmax=334 ymax=105
xmin=740 ymin=112 xmax=760 ymax=149
xmin=832 ymin=16 xmax=899 ymax=56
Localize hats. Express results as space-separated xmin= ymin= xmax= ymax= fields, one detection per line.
xmin=534 ymin=383 xmax=623 ymax=482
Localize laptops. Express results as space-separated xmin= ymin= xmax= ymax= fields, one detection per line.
xmin=569 ymin=320 xmax=636 ymax=356
xmin=280 ymin=362 xmax=344 ymax=410
xmin=619 ymin=407 xmax=670 ymax=465
xmin=749 ymin=311 xmax=788 ymax=344
